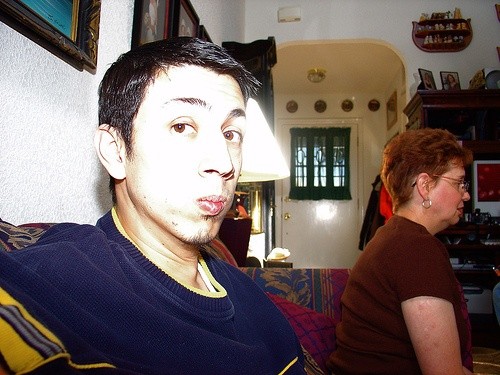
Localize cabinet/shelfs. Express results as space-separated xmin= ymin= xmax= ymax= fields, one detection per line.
xmin=402 ymin=91 xmax=500 ymax=350
xmin=415 ymin=19 xmax=469 ymax=48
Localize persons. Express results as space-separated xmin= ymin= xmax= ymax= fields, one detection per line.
xmin=0 ymin=36 xmax=306 ymax=375
xmin=447 ymin=74 xmax=457 ymax=90
xmin=425 ymin=73 xmax=433 ymax=89
xmin=327 ymin=128 xmax=474 ymax=375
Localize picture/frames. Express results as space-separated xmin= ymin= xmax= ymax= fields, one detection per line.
xmin=417 ymin=68 xmax=438 ymax=90
xmin=131 ymin=0 xmax=214 ymax=50
xmin=0 ymin=0 xmax=101 ymax=72
xmin=440 ymin=71 xmax=461 ymax=90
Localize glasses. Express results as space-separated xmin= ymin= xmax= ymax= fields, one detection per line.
xmin=411 ymin=174 xmax=471 ymax=191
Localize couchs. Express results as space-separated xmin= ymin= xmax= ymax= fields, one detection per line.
xmin=208 ymin=239 xmax=353 ymax=375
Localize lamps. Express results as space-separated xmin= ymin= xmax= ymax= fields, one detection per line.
xmin=239 ymin=98 xmax=290 ymax=181
